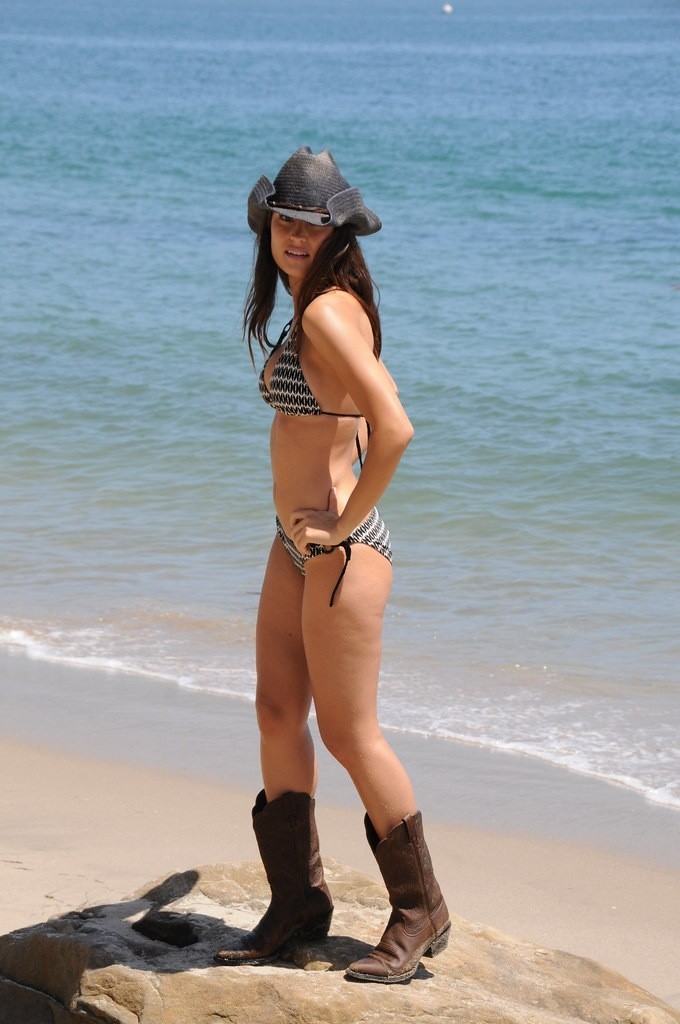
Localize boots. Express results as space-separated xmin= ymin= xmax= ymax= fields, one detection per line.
xmin=345 ymin=811 xmax=451 ymax=983
xmin=214 ymin=788 xmax=334 ymax=966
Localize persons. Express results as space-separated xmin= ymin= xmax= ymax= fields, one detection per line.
xmin=214 ymin=147 xmax=450 ymax=983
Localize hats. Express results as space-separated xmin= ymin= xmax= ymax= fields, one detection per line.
xmin=247 ymin=145 xmax=381 ymax=235
